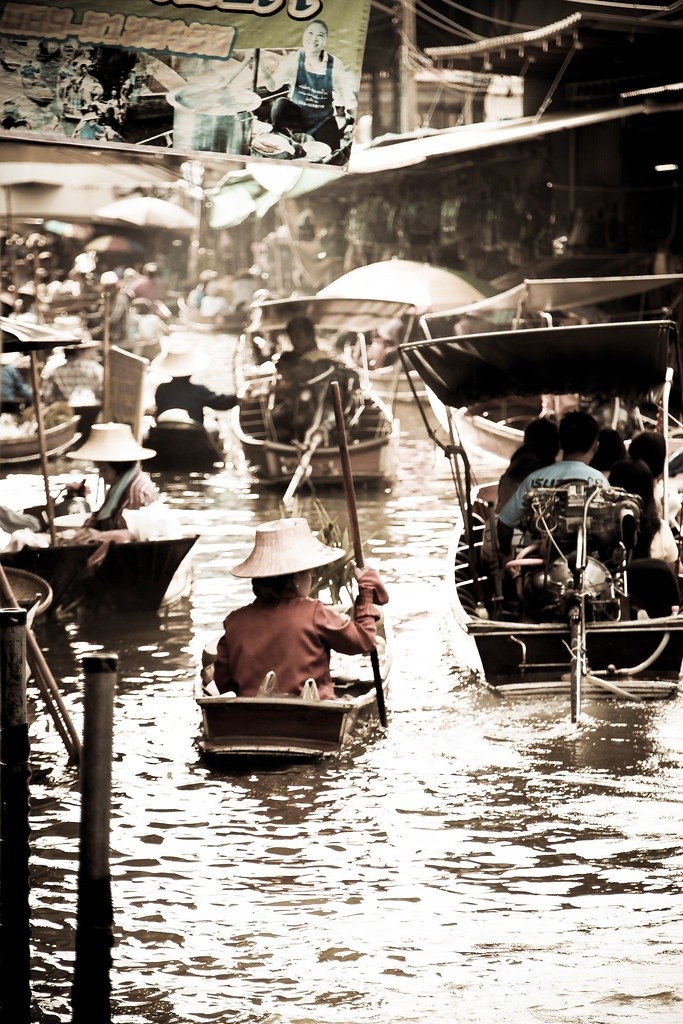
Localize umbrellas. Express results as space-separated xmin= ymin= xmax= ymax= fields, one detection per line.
xmin=314 ymin=259 xmax=501 ymax=416
xmin=85 ymin=234 xmax=144 ymax=256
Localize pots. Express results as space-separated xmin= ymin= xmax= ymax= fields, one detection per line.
xmin=165 ymin=79 xmax=263 ymax=157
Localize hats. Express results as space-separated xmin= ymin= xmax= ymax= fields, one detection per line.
xmin=230 ymin=518 xmax=347 ymax=578
xmin=66 ymin=421 xmax=158 ymax=462
xmin=100 ymin=271 xmax=117 ymax=286
xmin=163 ymin=339 xmax=199 ymax=378
xmin=62 ymin=331 xmax=102 ymax=349
xmin=198 ymin=268 xmax=217 ymax=282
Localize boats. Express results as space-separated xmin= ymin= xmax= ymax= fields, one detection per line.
xmin=230 ymin=285 xmax=415 ymax=492
xmin=56 ymin=44 xmax=186 ymax=119
xmin=136 ymin=408 xmax=226 ymax=469
xmin=0 ymin=315 xmax=200 ymax=614
xmin=198 ymin=601 xmax=390 ymax=756
xmin=19 ymin=72 xmax=54 ymax=107
xmin=19 ymin=292 xmax=252 ymax=361
xmin=396 ymin=275 xmax=683 ymax=701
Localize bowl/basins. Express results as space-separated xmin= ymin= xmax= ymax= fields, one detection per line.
xmin=251 ymin=135 xmax=290 ymax=156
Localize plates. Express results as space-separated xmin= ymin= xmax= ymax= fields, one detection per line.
xmin=303 ymin=140 xmax=333 ymax=164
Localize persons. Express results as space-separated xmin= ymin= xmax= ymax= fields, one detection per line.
xmin=0 ymin=364 xmax=32 ymax=413
xmin=213 ymin=518 xmax=389 ymax=700
xmin=42 ymin=339 xmax=104 ymax=401
xmin=66 ymin=421 xmax=161 ymax=531
xmin=270 ymin=19 xmax=358 ymax=152
xmin=271 ymin=316 xmax=334 ymax=428
xmin=155 ymin=341 xmax=238 ymax=425
xmin=495 ymin=411 xmax=682 ymax=571
xmin=17 ymin=262 xmax=229 ymax=363
